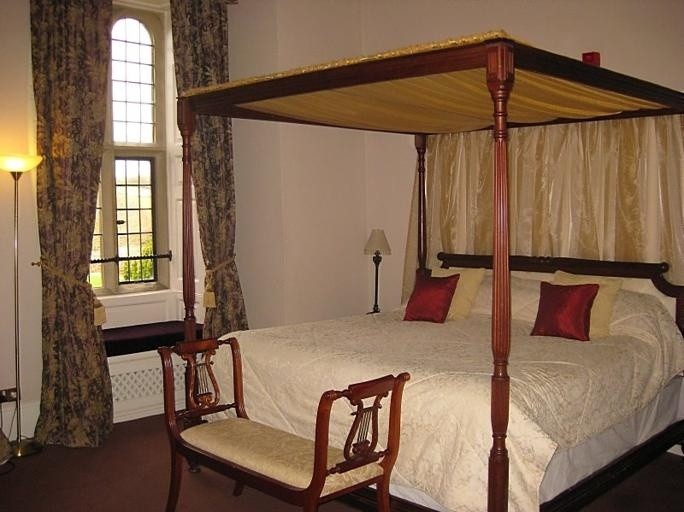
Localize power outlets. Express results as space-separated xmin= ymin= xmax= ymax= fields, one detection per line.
xmin=0 ymin=387 xmax=21 ymax=404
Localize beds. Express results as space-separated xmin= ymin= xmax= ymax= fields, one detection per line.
xmin=178 ymin=31 xmax=684 ymax=512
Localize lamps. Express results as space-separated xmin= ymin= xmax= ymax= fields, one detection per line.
xmin=364 ymin=228 xmax=392 ymax=315
xmin=0 ymin=154 xmax=44 ymax=458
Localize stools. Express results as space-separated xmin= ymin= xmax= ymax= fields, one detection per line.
xmin=157 ymin=337 xmax=410 ymax=512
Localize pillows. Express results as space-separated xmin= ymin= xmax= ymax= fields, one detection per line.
xmin=400 ymin=265 xmax=620 ymax=344
